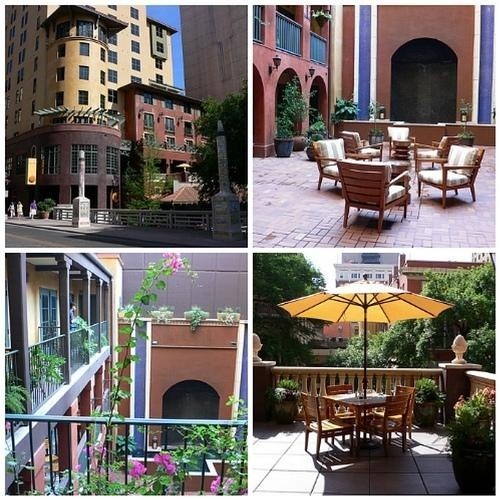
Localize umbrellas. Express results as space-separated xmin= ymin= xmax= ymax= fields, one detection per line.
xmin=277 ymin=272 xmax=455 ymax=438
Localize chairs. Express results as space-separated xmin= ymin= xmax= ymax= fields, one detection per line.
xmin=311 ymin=125 xmax=485 ymax=234
xmin=301 ymin=383 xmax=414 ymax=459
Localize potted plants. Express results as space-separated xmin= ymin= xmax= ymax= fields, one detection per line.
xmin=270 ymin=379 xmax=300 ymax=425
xmin=446 ymin=398 xmax=495 ymax=495
xmin=151 ymin=305 xmax=240 ymax=334
xmin=306 ymin=114 xmax=329 ymax=161
xmin=292 ymin=89 xmax=318 ymax=151
xmin=414 ymin=378 xmax=447 ymax=428
xmin=457 ymin=98 xmax=475 ymax=147
xmin=333 ymin=95 xmax=360 ymax=120
xmin=369 ymin=103 xmax=384 ymax=149
xmin=37 ymin=198 xmax=56 ymax=219
xmin=273 ymin=76 xmax=300 ymax=157
xmin=311 ymin=10 xmax=332 ymax=27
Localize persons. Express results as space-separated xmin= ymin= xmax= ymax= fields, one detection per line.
xmin=29 ymin=200 xmax=37 ymax=220
xmin=17 ymin=201 xmax=24 ymax=220
xmin=7 ymin=201 xmax=16 ymax=218
xmin=70 ymin=302 xmax=78 ymax=331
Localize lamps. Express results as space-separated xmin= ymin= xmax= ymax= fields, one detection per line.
xmin=269 ymin=55 xmax=281 ymax=74
xmin=305 ymin=66 xmax=315 ymax=81
